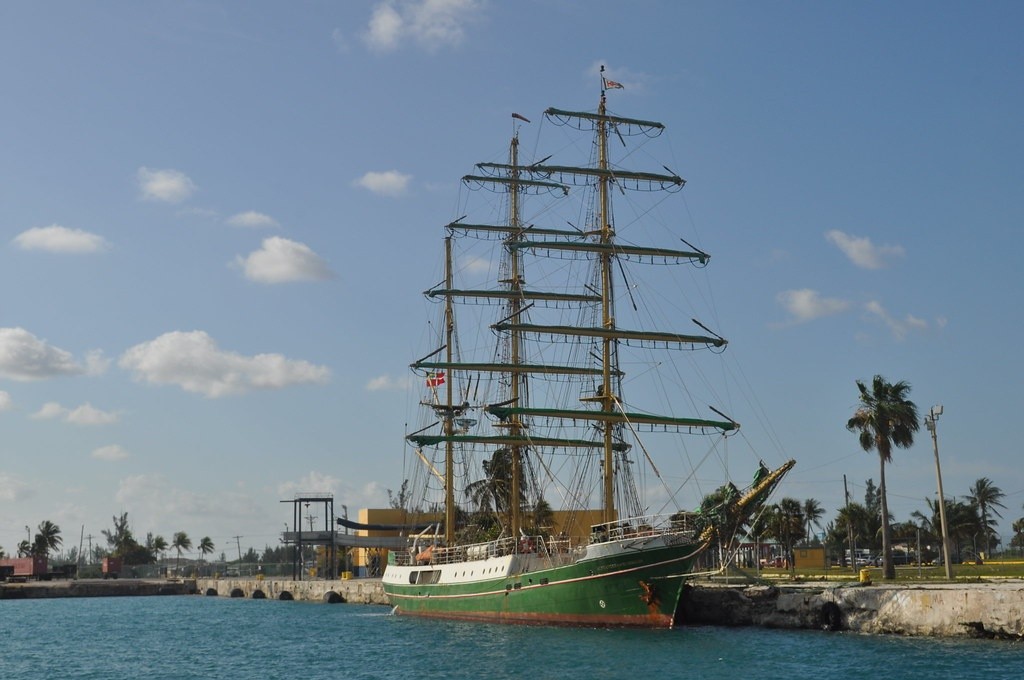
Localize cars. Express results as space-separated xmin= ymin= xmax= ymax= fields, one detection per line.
xmin=856 ymin=556 xmax=881 ymax=566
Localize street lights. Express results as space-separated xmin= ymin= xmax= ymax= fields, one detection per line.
xmin=924 ymin=404 xmax=955 ymax=580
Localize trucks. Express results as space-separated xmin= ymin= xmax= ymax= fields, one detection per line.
xmin=101 ymin=556 xmax=121 ymax=579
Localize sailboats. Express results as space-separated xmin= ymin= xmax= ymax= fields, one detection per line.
xmin=381 ymin=65 xmax=796 ymax=633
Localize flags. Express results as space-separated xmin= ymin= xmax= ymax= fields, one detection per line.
xmin=426 ymin=372 xmax=446 ymax=387
xmin=603 ymin=77 xmax=624 ymax=90
